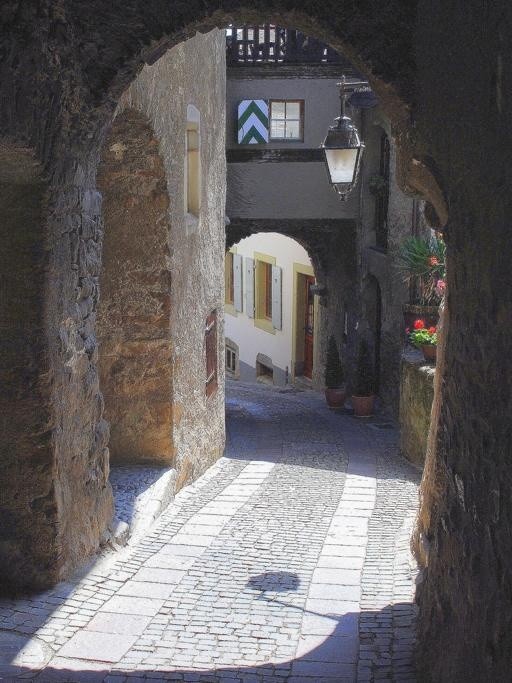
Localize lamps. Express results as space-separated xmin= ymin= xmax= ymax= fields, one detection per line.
xmin=319 ymin=75 xmax=368 ymax=202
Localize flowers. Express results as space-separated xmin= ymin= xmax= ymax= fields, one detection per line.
xmin=405 ymin=319 xmax=442 ymax=344
xmin=393 ymin=234 xmax=445 ymax=304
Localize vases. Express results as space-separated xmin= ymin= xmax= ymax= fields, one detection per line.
xmin=403 ymin=304 xmax=440 ymax=327
xmin=420 ymin=346 xmax=438 ymax=361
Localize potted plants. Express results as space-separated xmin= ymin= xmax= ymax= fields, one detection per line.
xmin=324 ymin=335 xmax=378 ymax=418
